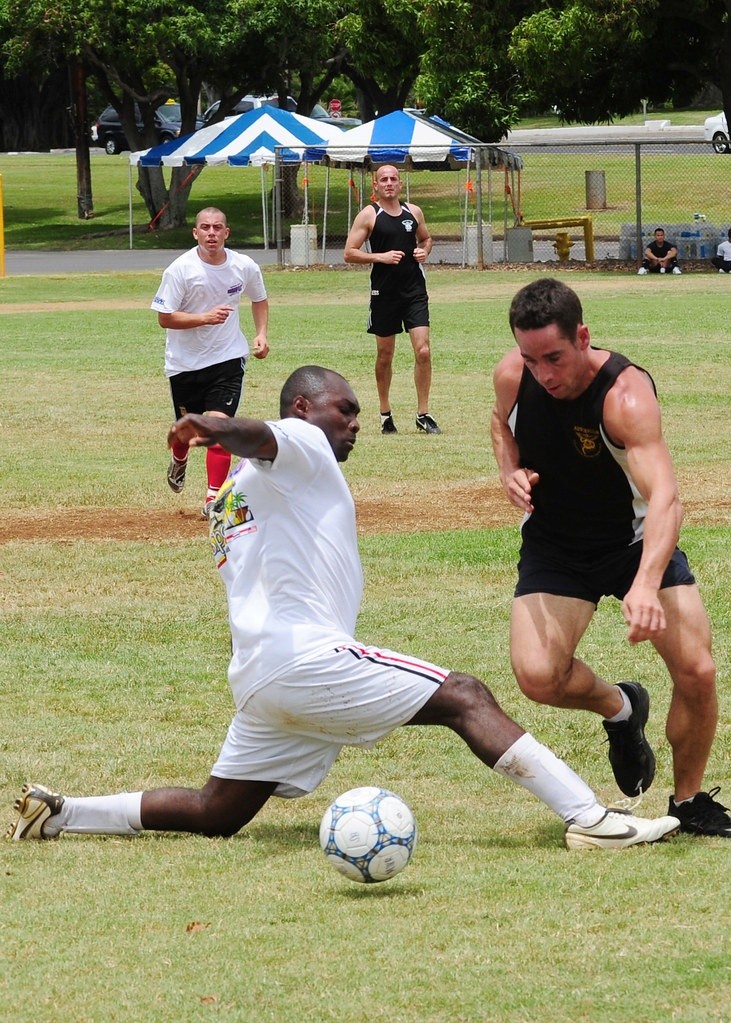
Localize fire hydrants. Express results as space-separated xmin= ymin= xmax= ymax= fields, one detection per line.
xmin=552 ymin=233 xmax=576 ymax=265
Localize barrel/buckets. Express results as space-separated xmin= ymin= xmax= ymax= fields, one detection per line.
xmin=289 ymin=223 xmax=318 ymax=264
xmin=506 ymin=226 xmax=534 ymax=263
xmin=466 ymin=224 xmax=493 ymax=265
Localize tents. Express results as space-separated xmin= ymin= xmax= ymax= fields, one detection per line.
xmin=275 ymin=107 xmax=524 ymax=271
xmin=127 ymin=104 xmax=348 ymax=250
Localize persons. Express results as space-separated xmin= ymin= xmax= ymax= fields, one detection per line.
xmin=343 ymin=165 xmax=442 ymax=435
xmin=711 ymin=228 xmax=731 ymax=274
xmin=0 ymin=365 xmax=680 ymax=853
xmin=637 ymin=228 xmax=682 ymax=275
xmin=150 ymin=206 xmax=271 ymax=518
xmin=490 ymin=279 xmax=731 ymax=838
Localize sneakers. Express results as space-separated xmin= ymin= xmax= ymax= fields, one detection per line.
xmin=565 ymin=786 xmax=680 ymax=850
xmin=666 ymin=786 xmax=731 ymax=836
xmin=415 ymin=413 xmax=440 ymax=434
xmin=167 ymin=459 xmax=187 ymax=493
xmin=673 ymin=266 xmax=681 ymax=274
xmin=200 ymin=498 xmax=215 ymax=518
xmin=637 ymin=267 xmax=647 ymax=274
xmin=599 ymin=680 xmax=655 ymax=798
xmin=6 ymin=782 xmax=63 ymax=841
xmin=380 ymin=411 xmax=397 ymax=434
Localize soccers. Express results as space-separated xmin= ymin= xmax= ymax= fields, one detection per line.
xmin=318 ymin=786 xmax=417 ymax=884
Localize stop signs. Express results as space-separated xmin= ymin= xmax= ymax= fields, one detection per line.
xmin=329 ymin=99 xmax=341 ymax=111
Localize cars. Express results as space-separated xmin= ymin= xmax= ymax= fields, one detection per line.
xmin=310 ymin=104 xmax=362 ymax=133
xmin=704 ymin=111 xmax=731 ymax=154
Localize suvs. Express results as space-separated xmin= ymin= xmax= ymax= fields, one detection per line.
xmin=200 ymin=94 xmax=301 ymax=122
xmin=91 ymin=99 xmax=207 ymax=155
